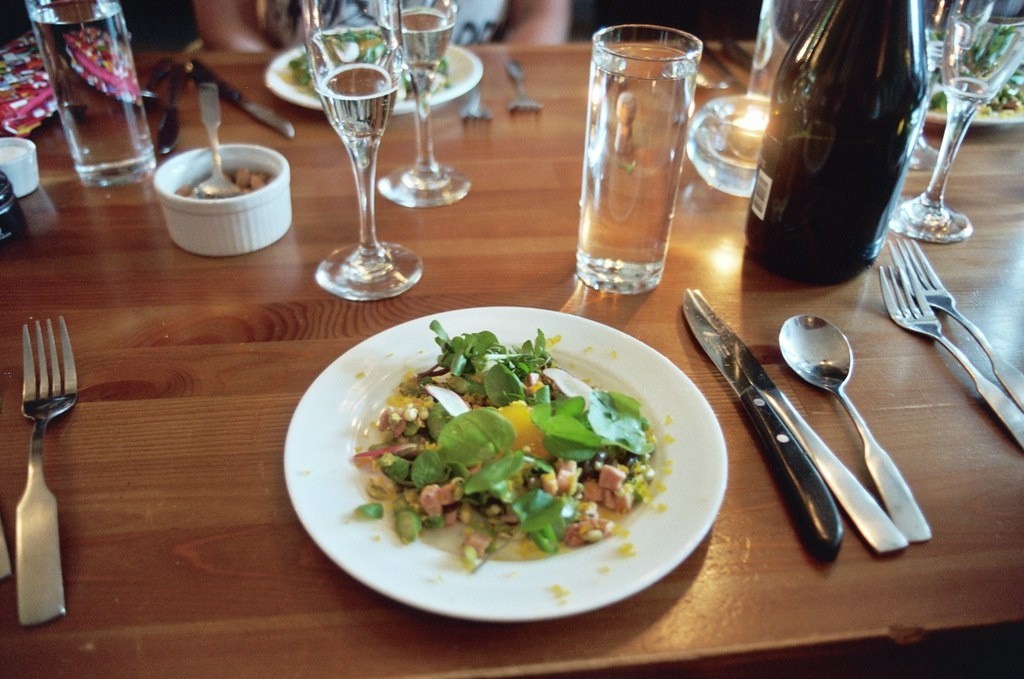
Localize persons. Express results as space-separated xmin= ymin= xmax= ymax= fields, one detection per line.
xmin=195 ymin=1 xmax=571 ymax=54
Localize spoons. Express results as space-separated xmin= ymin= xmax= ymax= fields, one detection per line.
xmin=778 ymin=314 xmax=932 ymax=542
xmin=142 ymin=59 xmax=172 ymax=113
xmin=194 ymin=81 xmax=242 ymax=202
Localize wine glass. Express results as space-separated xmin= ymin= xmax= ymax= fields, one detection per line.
xmin=300 ymin=0 xmax=422 ymax=301
xmin=378 ymin=0 xmax=473 ymax=207
xmin=887 ymin=0 xmax=1024 ymax=243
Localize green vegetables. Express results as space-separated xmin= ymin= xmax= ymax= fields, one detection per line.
xmin=922 ymin=15 xmax=1024 ymax=115
xmin=356 ymin=319 xmax=656 ymax=575
xmin=288 ymin=27 xmax=452 ymax=93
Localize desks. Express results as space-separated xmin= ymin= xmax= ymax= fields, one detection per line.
xmin=0 ymin=38 xmax=1024 ymax=679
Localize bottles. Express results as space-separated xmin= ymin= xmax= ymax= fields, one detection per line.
xmin=745 ymin=0 xmax=929 ymax=283
xmin=0 ymin=170 xmax=27 ymax=244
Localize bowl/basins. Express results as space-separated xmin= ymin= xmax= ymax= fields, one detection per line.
xmin=153 ymin=144 xmax=292 ymax=256
xmin=0 ymin=136 xmax=39 ymax=198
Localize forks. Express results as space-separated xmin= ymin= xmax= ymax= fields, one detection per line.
xmin=16 ymin=315 xmax=77 ymax=626
xmin=505 ymin=61 xmax=543 ymax=111
xmin=458 ymin=83 xmax=492 ymax=122
xmin=879 ymin=240 xmax=1024 ymax=452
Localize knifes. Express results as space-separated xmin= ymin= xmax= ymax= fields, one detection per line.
xmin=157 ymin=64 xmax=178 ymax=154
xmin=190 ymin=59 xmax=295 ymax=139
xmin=681 ymin=288 xmax=909 ymax=561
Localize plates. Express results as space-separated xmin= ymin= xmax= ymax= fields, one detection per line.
xmin=264 ymin=42 xmax=484 ymax=115
xmin=284 ymin=307 xmax=728 ymax=622
xmin=919 ymin=25 xmax=1024 ymax=129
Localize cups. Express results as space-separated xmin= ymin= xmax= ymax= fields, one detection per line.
xmin=23 ymin=0 xmax=156 ymax=188
xmin=576 ymin=23 xmax=702 ymax=294
xmin=686 ymin=0 xmax=825 ymax=197
